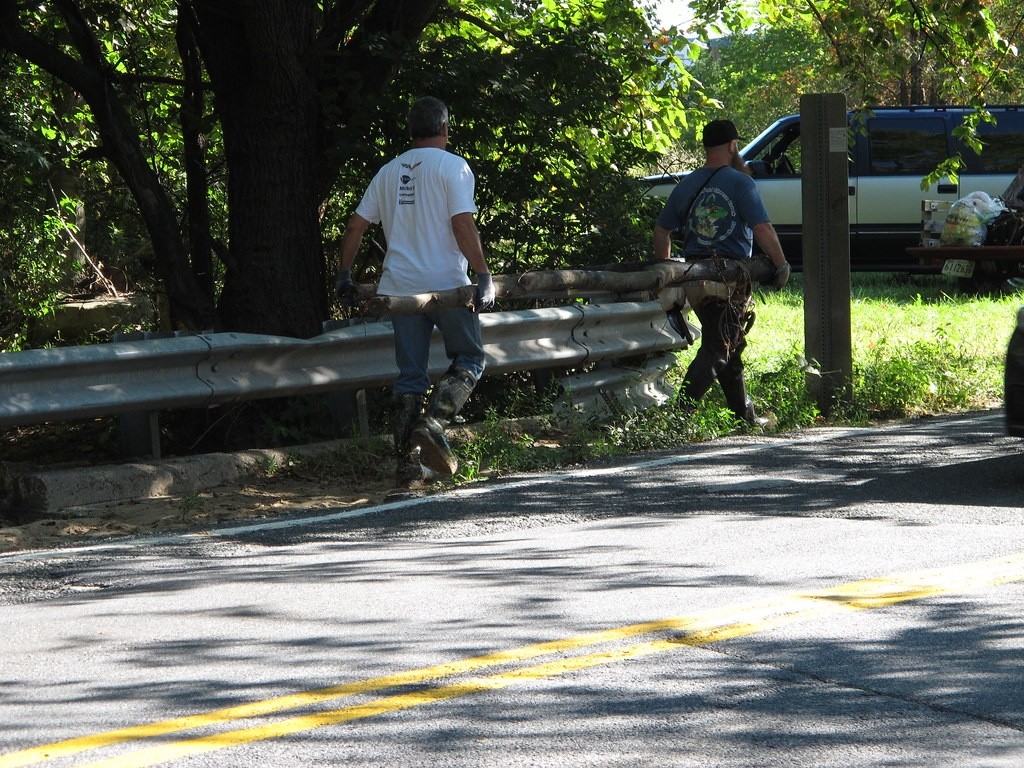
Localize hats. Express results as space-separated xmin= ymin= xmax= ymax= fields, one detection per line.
xmin=703 ymin=120 xmax=747 ymax=146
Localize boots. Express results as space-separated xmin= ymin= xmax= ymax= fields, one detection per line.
xmin=390 ymin=390 xmax=444 ymax=489
xmin=676 ymin=365 xmax=713 ymax=421
xmin=717 ymin=376 xmax=774 ymax=428
xmin=412 ymin=363 xmax=476 ymax=472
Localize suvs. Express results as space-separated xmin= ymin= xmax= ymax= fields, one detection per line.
xmin=636 ymin=105 xmax=1024 ymax=274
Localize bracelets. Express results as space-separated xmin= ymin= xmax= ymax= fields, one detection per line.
xmin=774 ymin=264 xmax=790 ymax=272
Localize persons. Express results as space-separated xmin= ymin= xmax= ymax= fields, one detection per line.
xmin=652 ymin=117 xmax=794 ymax=431
xmin=333 ymin=97 xmax=499 ymax=488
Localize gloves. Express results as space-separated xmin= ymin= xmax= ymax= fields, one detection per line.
xmin=774 ymin=263 xmax=792 ymax=291
xmin=474 ymin=273 xmax=496 ymax=315
xmin=335 ymin=272 xmax=362 ymax=308
